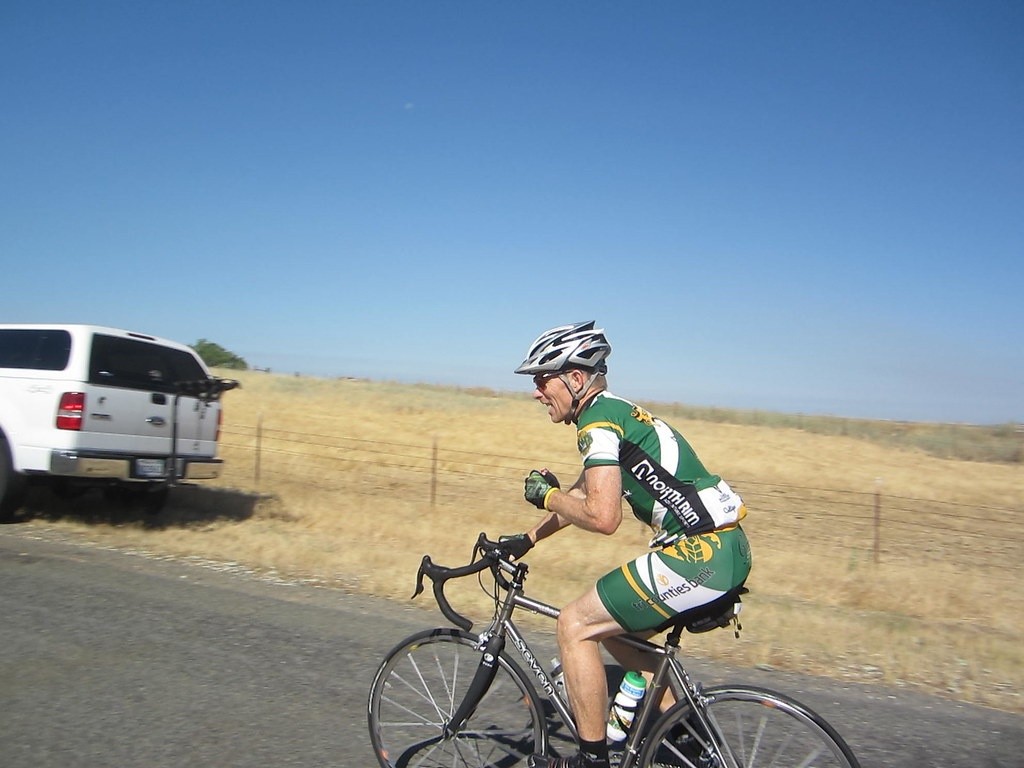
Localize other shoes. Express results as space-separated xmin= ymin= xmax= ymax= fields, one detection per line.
xmin=527 ymin=750 xmax=607 ymax=768
xmin=655 ymin=747 xmax=715 ymax=768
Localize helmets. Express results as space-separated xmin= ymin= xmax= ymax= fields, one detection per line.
xmin=514 ymin=320 xmax=611 ymax=375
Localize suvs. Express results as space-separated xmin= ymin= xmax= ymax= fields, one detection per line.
xmin=0 ymin=324 xmax=239 ymax=519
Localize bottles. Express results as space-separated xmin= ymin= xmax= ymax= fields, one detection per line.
xmin=550 ymin=657 xmax=574 ymax=716
xmin=607 ymin=671 xmax=646 ymax=741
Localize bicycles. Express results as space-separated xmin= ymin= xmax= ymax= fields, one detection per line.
xmin=368 ymin=534 xmax=862 ymax=767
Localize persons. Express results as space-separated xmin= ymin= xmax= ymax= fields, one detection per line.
xmin=498 ymin=321 xmax=751 ymax=768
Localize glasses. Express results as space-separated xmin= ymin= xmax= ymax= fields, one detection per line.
xmin=532 ymin=369 xmax=583 ymax=388
